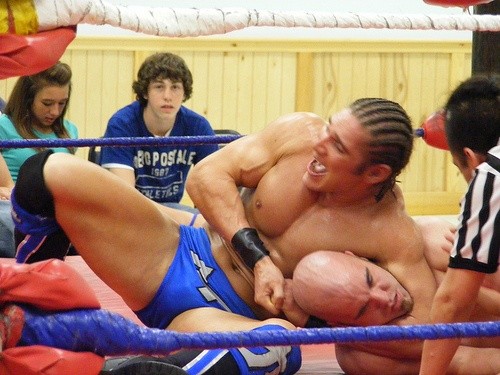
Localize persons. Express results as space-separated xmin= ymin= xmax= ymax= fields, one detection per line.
xmin=0 ymin=60 xmax=89 ymax=201
xmin=0 ymin=79 xmax=500 ymax=375
xmin=103 ymin=52 xmax=218 ymax=203
